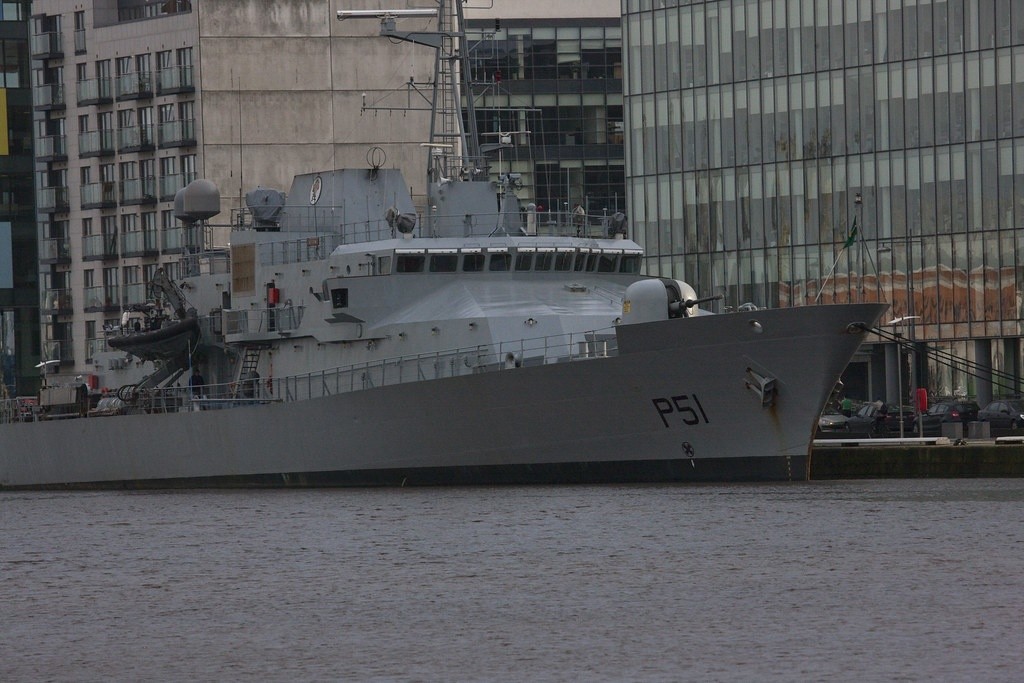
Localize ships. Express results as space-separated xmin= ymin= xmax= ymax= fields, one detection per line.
xmin=2 ymin=0 xmax=891 ymax=493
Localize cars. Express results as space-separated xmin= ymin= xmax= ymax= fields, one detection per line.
xmin=975 ymin=398 xmax=1024 ymax=431
xmin=817 ymin=401 xmax=850 ymax=433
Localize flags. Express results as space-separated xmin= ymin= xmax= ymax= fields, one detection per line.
xmin=840 ymin=215 xmax=860 ymax=250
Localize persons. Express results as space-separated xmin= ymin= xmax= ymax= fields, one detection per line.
xmin=189 ymin=370 xmax=203 ymax=396
xmin=840 ymin=396 xmax=852 ymax=417
xmin=875 ymin=397 xmax=883 ymax=413
xmin=571 ymin=202 xmax=586 ymax=237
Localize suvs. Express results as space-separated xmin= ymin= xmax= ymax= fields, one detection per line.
xmin=844 ymin=402 xmax=916 ymax=439
xmin=910 ymin=397 xmax=981 ymax=434
